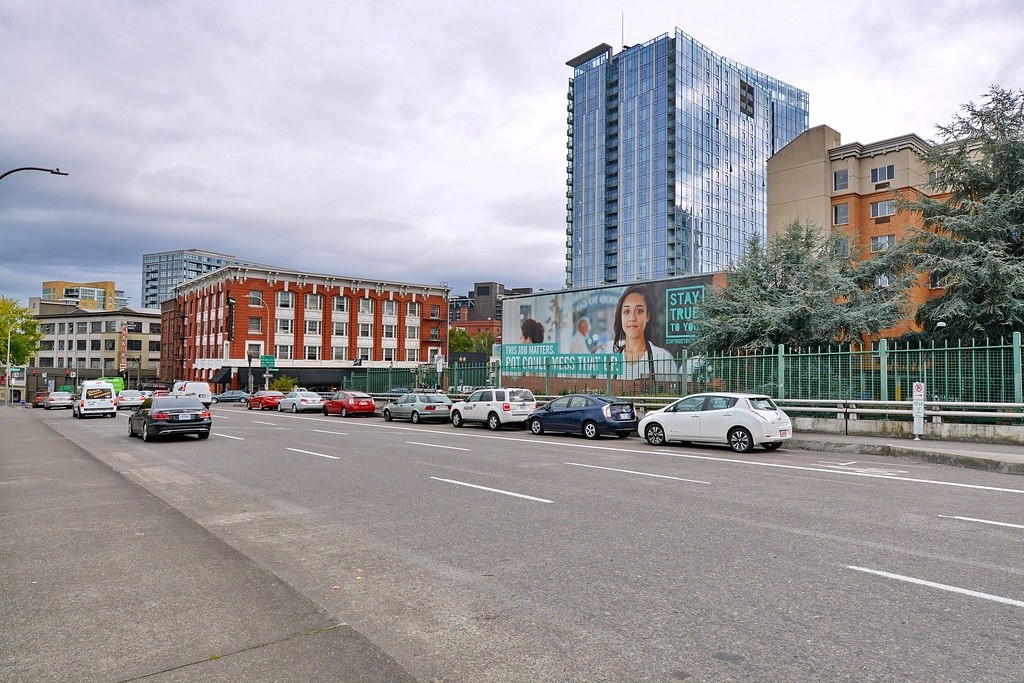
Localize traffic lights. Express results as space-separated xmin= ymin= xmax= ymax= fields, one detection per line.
xmin=33 ymin=370 xmax=37 ymax=377
xmin=4 ymin=371 xmax=7 ymax=376
xmin=66 ymin=370 xmax=69 ymax=376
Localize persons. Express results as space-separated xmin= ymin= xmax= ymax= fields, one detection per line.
xmin=594 ymin=286 xmax=677 ymax=380
xmin=520 ymin=319 xmax=545 ymax=344
xmin=570 ymin=317 xmax=605 ymax=354
xmin=137 ymin=383 xmax=143 ymax=391
xmin=421 ymin=381 xmax=427 ymax=388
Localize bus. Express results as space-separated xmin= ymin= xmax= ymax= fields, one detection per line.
xmin=96 ymin=377 xmax=126 ymax=397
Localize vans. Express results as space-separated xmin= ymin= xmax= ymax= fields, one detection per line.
xmin=70 ymin=381 xmax=118 ymax=419
xmin=173 ymin=381 xmax=212 ymax=408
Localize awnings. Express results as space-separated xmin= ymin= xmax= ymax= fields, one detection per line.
xmin=238 ymin=368 xmax=299 ymax=384
xmin=297 ymin=368 xmax=367 ymax=385
xmin=211 ymin=368 xmax=230 ymax=383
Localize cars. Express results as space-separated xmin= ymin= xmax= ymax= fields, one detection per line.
xmin=446 ymin=386 xmax=494 ymax=394
xmin=115 ymin=390 xmax=146 ymax=409
xmin=141 ymin=391 xmax=153 ymax=398
xmin=382 ymin=392 xmax=455 ymax=425
xmin=154 ymin=390 xmax=170 ymax=398
xmin=209 ymin=390 xmax=253 ymax=404
xmin=128 ymin=394 xmax=213 ymax=442
xmin=30 ymin=392 xmax=50 ymax=408
xmin=322 ymin=390 xmax=376 ymax=417
xmin=637 ymin=391 xmax=792 ymax=452
xmin=44 ymin=392 xmax=75 ymax=410
xmin=386 ymin=387 xmax=410 ymax=393
xmin=71 ymin=394 xmax=77 ymax=399
xmin=277 ymin=392 xmax=325 ymax=413
xmin=247 ymin=389 xmax=285 ymax=410
xmin=526 ymin=393 xmax=639 ymax=439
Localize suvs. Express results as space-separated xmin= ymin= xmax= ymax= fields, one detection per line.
xmin=449 ymin=386 xmax=538 ymax=431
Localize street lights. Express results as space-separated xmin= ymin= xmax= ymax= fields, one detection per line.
xmin=7 ymin=319 xmax=39 ymax=406
xmin=459 ymin=356 xmax=467 ymax=393
xmin=241 ymin=295 xmax=270 ymax=389
xmin=246 ymin=349 xmax=254 ymax=394
